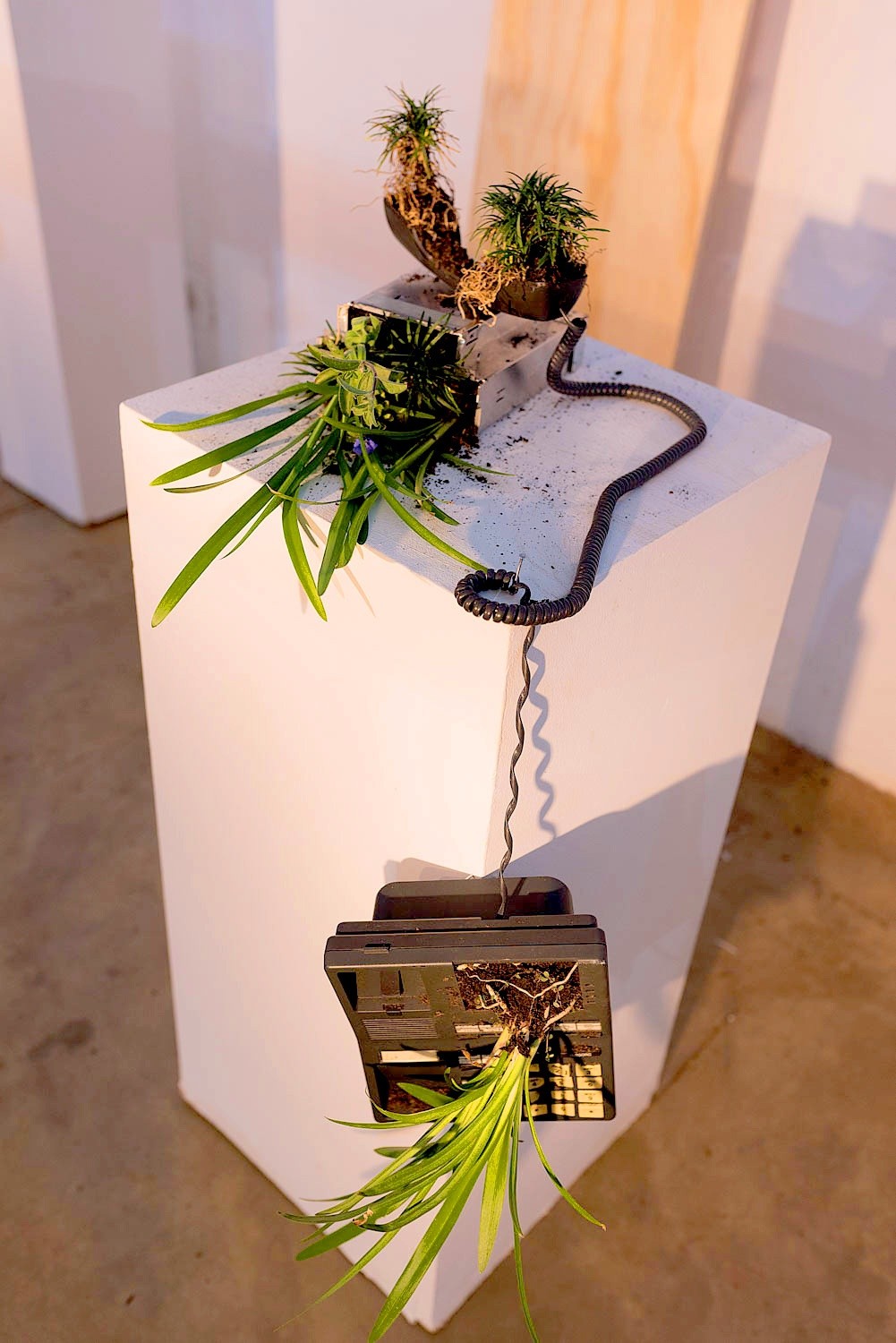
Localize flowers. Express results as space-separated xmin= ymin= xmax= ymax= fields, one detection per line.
xmin=141 ymin=308 xmax=521 ymax=624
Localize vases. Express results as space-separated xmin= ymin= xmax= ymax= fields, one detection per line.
xmin=338 ymin=264 xmax=574 ymax=437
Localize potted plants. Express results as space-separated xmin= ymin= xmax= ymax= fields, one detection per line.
xmin=280 ymin=882 xmax=619 ymax=1343
xmin=376 ymin=92 xmax=587 ymax=320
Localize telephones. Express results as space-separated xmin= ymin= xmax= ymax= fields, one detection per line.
xmin=384 ymin=191 xmax=588 ymax=322
